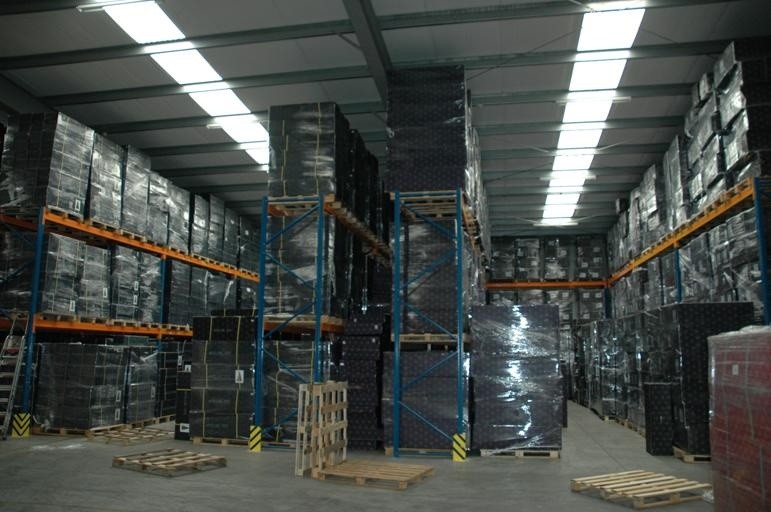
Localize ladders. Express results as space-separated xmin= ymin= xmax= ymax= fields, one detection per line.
xmin=1 ymin=334 xmax=25 ymax=437
